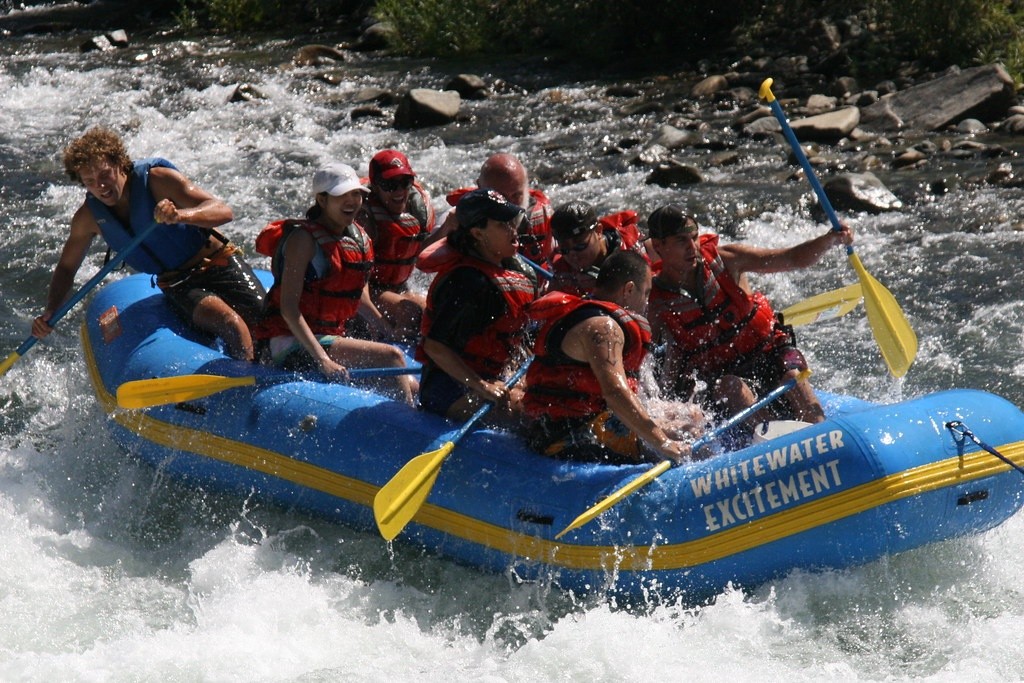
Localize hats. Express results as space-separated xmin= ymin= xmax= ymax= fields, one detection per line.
xmin=313 ymin=161 xmax=371 ymax=196
xmin=550 ymin=201 xmax=599 ymax=240
xmin=455 ymin=187 xmax=521 ymax=228
xmin=369 ymin=150 xmax=416 ymax=182
xmin=639 ymin=202 xmax=698 ymax=243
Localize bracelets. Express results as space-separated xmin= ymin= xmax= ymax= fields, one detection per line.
xmin=661 ymin=439 xmax=671 ymax=448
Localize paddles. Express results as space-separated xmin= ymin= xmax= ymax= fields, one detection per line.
xmin=554 ymin=368 xmax=810 ymax=540
xmin=116 ymin=364 xmax=422 ymax=410
xmin=1 ymin=217 xmax=163 ymax=374
xmin=781 ymin=282 xmax=862 ymax=325
xmin=372 ymin=352 xmax=534 ymax=542
xmin=758 ymin=76 xmax=917 ymax=379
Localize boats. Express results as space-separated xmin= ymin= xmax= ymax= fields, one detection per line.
xmin=81 ymin=265 xmax=1024 ymax=606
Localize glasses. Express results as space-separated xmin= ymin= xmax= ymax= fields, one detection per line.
xmin=554 ymin=233 xmax=592 ymax=255
xmin=374 ymin=176 xmax=413 ymax=192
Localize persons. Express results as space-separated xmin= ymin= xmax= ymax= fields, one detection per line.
xmin=33 ymin=129 xmax=264 ymax=363
xmin=419 ymin=155 xmax=558 ymax=252
xmin=266 ymin=162 xmax=417 ymax=407
xmin=639 ymin=204 xmax=853 ymax=428
xmin=546 ymin=200 xmax=661 ymax=304
xmin=358 ymin=151 xmax=435 ymax=329
xmin=412 ymin=187 xmax=538 ymax=426
xmin=520 ymin=248 xmax=711 ymax=469
